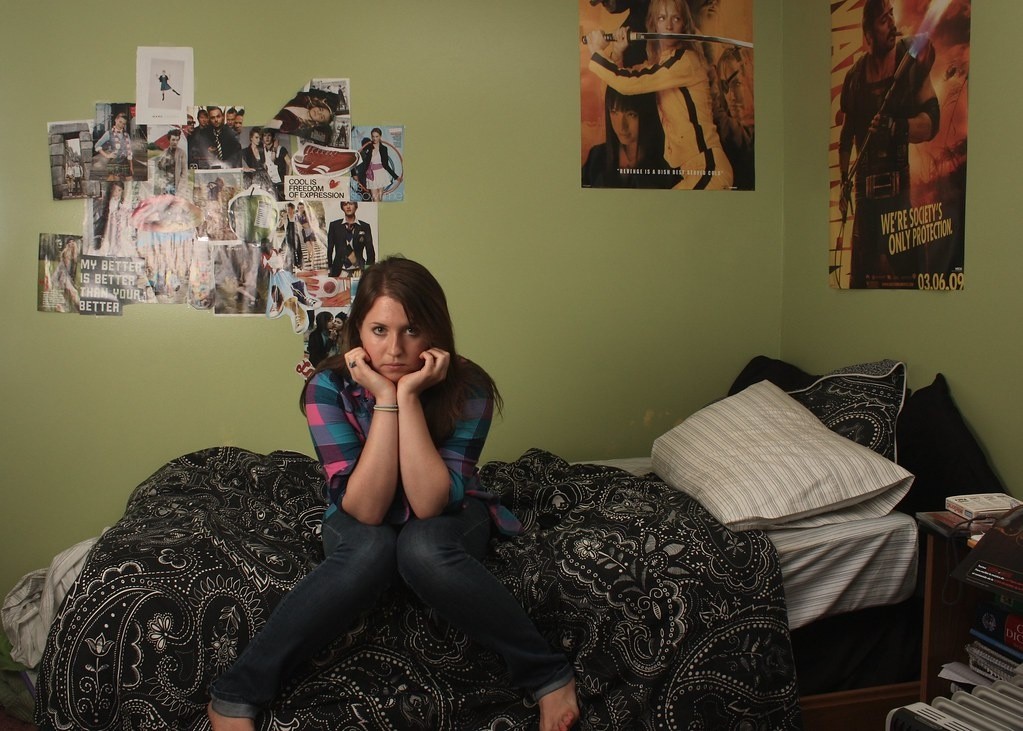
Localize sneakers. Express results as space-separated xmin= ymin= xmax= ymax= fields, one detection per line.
xmin=292 ymin=145 xmax=360 ymax=177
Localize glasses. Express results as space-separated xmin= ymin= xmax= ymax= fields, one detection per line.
xmin=187 ymin=121 xmax=196 ymax=125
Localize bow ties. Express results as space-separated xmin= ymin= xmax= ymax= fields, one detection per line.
xmin=265 ymin=145 xmax=273 ymax=152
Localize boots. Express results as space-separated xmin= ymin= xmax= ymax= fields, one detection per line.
xmin=284 ymin=296 xmax=307 ymax=333
xmin=172 ymin=89 xmax=180 ymax=95
xmin=268 ymin=285 xmax=285 ymax=318
xmin=290 ymin=280 xmax=323 ymax=310
xmin=162 ymin=93 xmax=164 ymax=101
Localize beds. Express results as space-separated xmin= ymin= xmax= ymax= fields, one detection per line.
xmin=50 ymin=455 xmax=916 ymax=731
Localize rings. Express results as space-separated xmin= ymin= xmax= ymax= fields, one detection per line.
xmin=348 ymin=360 xmax=356 ymax=369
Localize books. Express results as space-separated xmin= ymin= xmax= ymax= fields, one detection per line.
xmin=945 ymin=493 xmax=1023 ymax=694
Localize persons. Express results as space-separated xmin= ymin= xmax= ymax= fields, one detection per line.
xmin=208 ymin=253 xmax=579 ymax=731
xmin=582 ymin=0 xmax=754 ymax=190
xmin=839 ymin=0 xmax=940 ymax=289
xmin=48 ymin=70 xmax=404 ymax=370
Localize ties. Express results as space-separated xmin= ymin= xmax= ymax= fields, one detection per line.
xmin=212 ymin=128 xmax=223 ymax=161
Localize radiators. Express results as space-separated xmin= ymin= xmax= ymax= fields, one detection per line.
xmin=885 ymin=675 xmax=1023 ymax=731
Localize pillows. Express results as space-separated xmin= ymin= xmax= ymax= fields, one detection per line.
xmin=785 ymin=359 xmax=906 ymax=472
xmin=652 ymin=380 xmax=915 ymax=531
xmin=727 ymin=355 xmax=823 ymax=399
xmin=892 ymin=374 xmax=1013 ymax=518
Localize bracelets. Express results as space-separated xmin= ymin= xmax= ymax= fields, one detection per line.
xmin=893 ymin=118 xmax=910 ymax=143
xmin=840 ymin=172 xmax=848 ymax=188
xmin=373 ymin=405 xmax=398 ymax=411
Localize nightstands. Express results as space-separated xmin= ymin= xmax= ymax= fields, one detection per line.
xmin=918 ymin=526 xmax=1023 ymax=702
